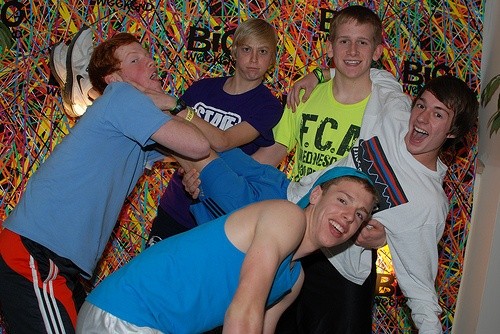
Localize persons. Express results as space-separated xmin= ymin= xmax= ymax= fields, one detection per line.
xmin=0 ymin=33 xmax=210 ymax=334
xmin=77 ymin=167 xmax=380 ymax=334
xmin=50 ymin=24 xmax=479 ymax=334
xmin=125 ymin=19 xmax=284 ymax=250
xmin=178 ymin=5 xmax=388 ymax=334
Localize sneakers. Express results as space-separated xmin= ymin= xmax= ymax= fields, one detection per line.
xmin=50 ymin=42 xmax=87 ymax=117
xmin=66 ymin=26 xmax=95 ymax=106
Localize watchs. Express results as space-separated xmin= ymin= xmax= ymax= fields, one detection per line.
xmin=171 ymin=99 xmax=187 ymax=116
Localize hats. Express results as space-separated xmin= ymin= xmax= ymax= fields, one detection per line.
xmin=296 ymin=166 xmax=376 ymax=208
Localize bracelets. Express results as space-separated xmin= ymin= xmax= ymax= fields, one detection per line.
xmin=313 ymin=67 xmax=325 ymax=83
xmin=185 ymin=106 xmax=195 ymax=121
